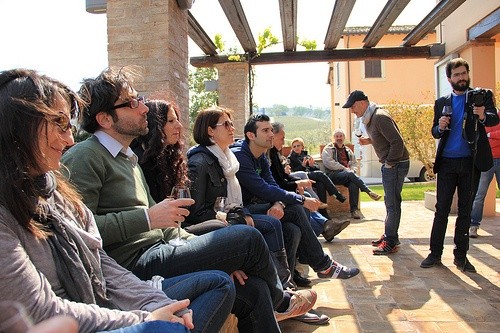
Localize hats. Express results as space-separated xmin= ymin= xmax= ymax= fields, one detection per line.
xmin=342 ymin=90 xmax=368 ymax=108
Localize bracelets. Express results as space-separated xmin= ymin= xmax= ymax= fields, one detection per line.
xmin=275 ymin=200 xmax=286 ymax=209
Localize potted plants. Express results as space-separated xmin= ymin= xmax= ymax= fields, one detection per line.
xmin=384 ymin=89 xmax=456 ymax=213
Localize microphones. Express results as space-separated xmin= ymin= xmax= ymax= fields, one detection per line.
xmin=474 ymin=94 xmax=484 ymax=119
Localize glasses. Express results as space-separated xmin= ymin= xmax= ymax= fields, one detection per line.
xmin=43 ymin=115 xmax=77 ymax=135
xmin=214 ymin=120 xmax=234 ymax=127
xmin=107 ymin=95 xmax=144 ymax=109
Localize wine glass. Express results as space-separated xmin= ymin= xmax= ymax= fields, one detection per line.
xmin=170 ymin=186 xmax=191 ymax=245
xmin=442 ymin=105 xmax=453 ymax=131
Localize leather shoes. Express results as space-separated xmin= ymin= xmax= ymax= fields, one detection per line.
xmin=294 ymin=309 xmax=330 ymax=324
xmin=322 ymin=220 xmax=350 ymax=241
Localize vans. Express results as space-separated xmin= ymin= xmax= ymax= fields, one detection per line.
xmin=352 ymin=103 xmax=446 ymax=184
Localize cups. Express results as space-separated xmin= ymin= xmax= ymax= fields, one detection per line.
xmin=213 ymin=197 xmax=230 ymax=216
xmin=356 ymin=128 xmax=362 ymax=138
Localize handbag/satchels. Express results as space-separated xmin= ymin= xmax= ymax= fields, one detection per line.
xmin=226 ymin=207 xmax=247 ymax=225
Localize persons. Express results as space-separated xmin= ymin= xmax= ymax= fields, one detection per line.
xmin=129 ymin=101 xmax=360 ymax=325
xmin=467 ymin=89 xmax=500 ymax=238
xmin=420 ymin=57 xmax=499 ymax=274
xmin=320 ymin=131 xmax=382 ymax=220
xmin=0 ymin=69 xmax=237 ymax=333
xmin=59 ymin=71 xmax=317 ymax=333
xmin=342 ymin=90 xmax=410 ymax=255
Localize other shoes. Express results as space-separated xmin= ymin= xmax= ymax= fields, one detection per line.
xmin=336 ymin=194 xmax=346 ymax=203
xmin=351 ymin=209 xmax=364 ymax=219
xmin=320 ymin=212 xmax=331 ymax=220
xmin=370 ymin=192 xmax=382 ymax=200
xmin=318 ymin=201 xmax=328 ymax=208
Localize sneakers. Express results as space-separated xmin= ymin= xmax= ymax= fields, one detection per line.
xmin=373 ymin=240 xmax=398 ymax=254
xmin=454 ymin=256 xmax=476 ymax=272
xmin=293 ymin=271 xmax=311 ymax=286
xmin=317 ymin=259 xmax=360 ymax=279
xmin=420 ymin=255 xmax=441 ymax=267
xmin=469 ymin=226 xmax=479 ymax=237
xmin=372 ymin=234 xmax=400 ymax=246
xmin=273 ymin=290 xmax=317 ymax=322
